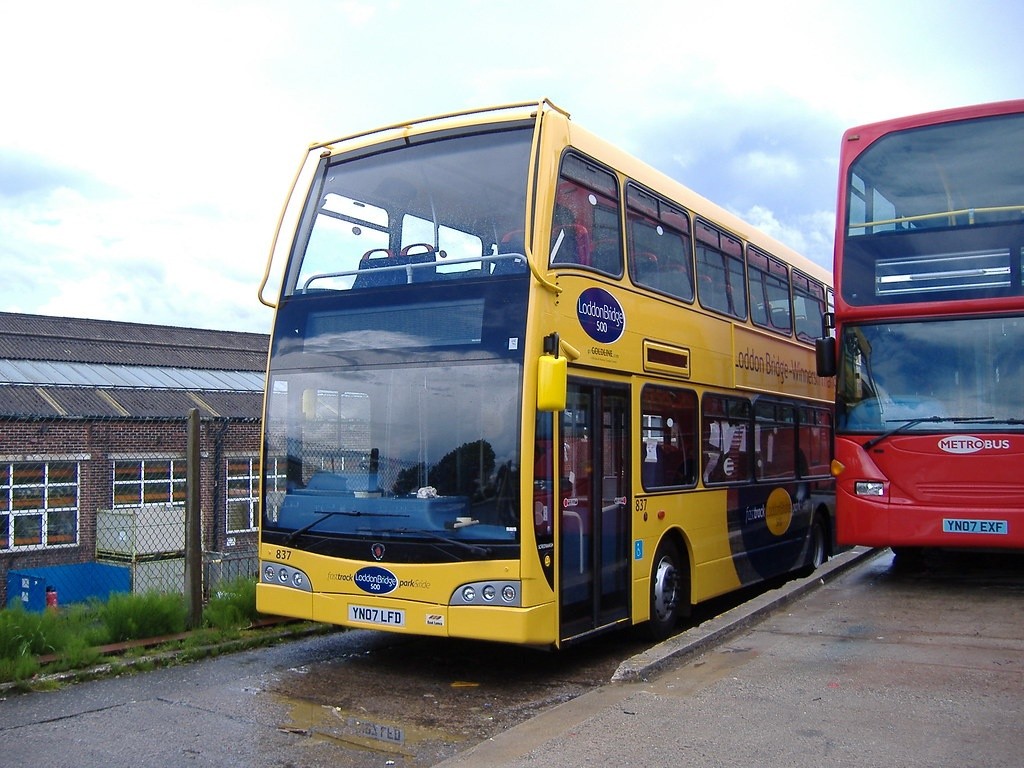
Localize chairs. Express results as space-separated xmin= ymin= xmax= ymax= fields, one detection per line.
xmin=353 ymin=244 xmax=437 ymax=290
xmin=492 ymin=222 xmax=821 ymax=340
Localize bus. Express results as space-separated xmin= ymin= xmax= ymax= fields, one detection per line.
xmin=255 ymin=98 xmax=864 ymax=657
xmin=814 ymin=95 xmax=1023 ymax=563
